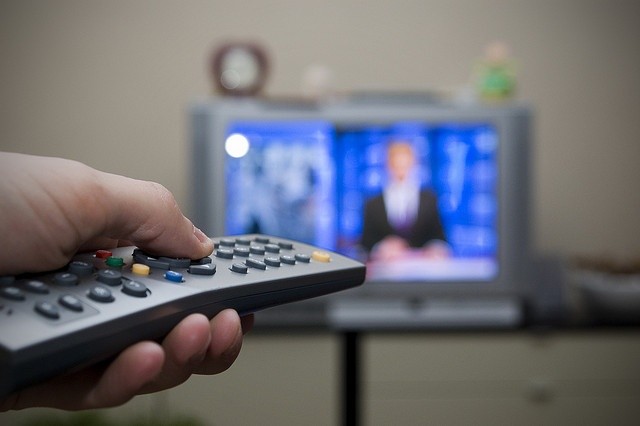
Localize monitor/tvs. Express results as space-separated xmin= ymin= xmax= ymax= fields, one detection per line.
xmin=189 ymin=86 xmax=533 ymax=331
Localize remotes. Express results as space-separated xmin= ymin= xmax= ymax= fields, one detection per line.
xmin=0 ymin=233 xmax=366 ymax=371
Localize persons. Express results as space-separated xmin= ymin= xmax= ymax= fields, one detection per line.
xmin=0 ymin=150 xmax=254 ymax=412
